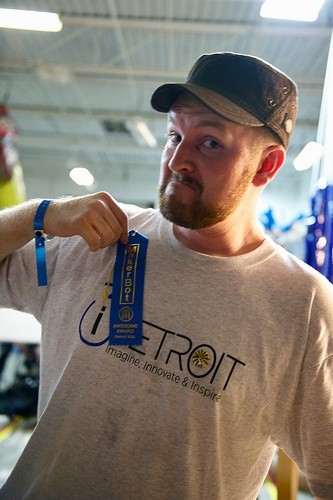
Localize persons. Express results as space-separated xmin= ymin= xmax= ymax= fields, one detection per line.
xmin=0 ymin=49 xmax=333 ymax=500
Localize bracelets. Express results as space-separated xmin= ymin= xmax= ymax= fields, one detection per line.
xmin=33 ymin=198 xmax=56 ymax=286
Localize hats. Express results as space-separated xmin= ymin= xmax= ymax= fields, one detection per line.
xmin=151 ymin=51 xmax=299 ymax=147
